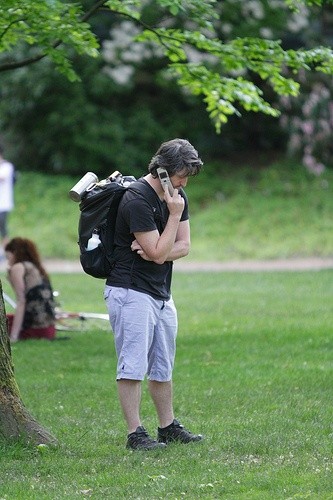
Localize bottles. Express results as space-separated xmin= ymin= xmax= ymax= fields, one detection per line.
xmin=87 ymin=228 xmax=102 ymax=252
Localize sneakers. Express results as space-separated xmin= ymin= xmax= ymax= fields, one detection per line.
xmin=126 ymin=425 xmax=168 ymax=451
xmin=157 ymin=419 xmax=204 ymax=444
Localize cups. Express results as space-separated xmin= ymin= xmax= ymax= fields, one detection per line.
xmin=69 ymin=172 xmax=99 ymax=202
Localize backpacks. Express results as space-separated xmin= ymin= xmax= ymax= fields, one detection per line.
xmin=77 ymin=170 xmax=162 ymax=279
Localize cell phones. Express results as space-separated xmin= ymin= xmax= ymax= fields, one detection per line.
xmin=157 ymin=167 xmax=175 ymax=197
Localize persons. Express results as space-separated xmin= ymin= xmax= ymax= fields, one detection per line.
xmin=104 ymin=138 xmax=203 ymax=452
xmin=0 ymin=148 xmax=14 ymax=265
xmin=4 ymin=236 xmax=55 ymax=342
